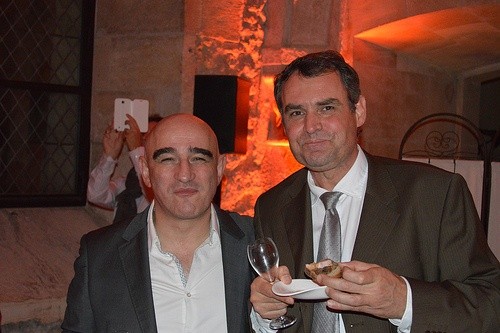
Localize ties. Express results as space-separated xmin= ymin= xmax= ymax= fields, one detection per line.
xmin=313 ymin=190 xmax=343 ymax=333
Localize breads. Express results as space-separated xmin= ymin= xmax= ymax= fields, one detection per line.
xmin=304 ymin=259 xmax=343 ymax=284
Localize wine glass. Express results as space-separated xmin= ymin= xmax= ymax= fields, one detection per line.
xmin=247 ymin=238 xmax=298 ymax=329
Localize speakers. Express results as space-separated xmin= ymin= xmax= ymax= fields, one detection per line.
xmin=193 ymin=75 xmax=249 ymax=155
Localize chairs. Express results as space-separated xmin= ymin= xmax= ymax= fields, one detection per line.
xmin=398 ymin=113 xmax=490 ymax=238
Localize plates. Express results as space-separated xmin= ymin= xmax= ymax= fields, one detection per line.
xmin=272 ymin=278 xmax=333 ymax=299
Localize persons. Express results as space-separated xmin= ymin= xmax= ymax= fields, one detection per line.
xmin=247 ymin=49 xmax=500 ymax=333
xmin=87 ymin=113 xmax=164 ymax=225
xmin=61 ymin=113 xmax=260 ymax=333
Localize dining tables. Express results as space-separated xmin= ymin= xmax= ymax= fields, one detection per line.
xmin=402 ymin=157 xmax=500 ymax=262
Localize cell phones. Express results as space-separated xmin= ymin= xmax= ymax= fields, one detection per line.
xmin=114 ymin=98 xmax=149 ymax=133
